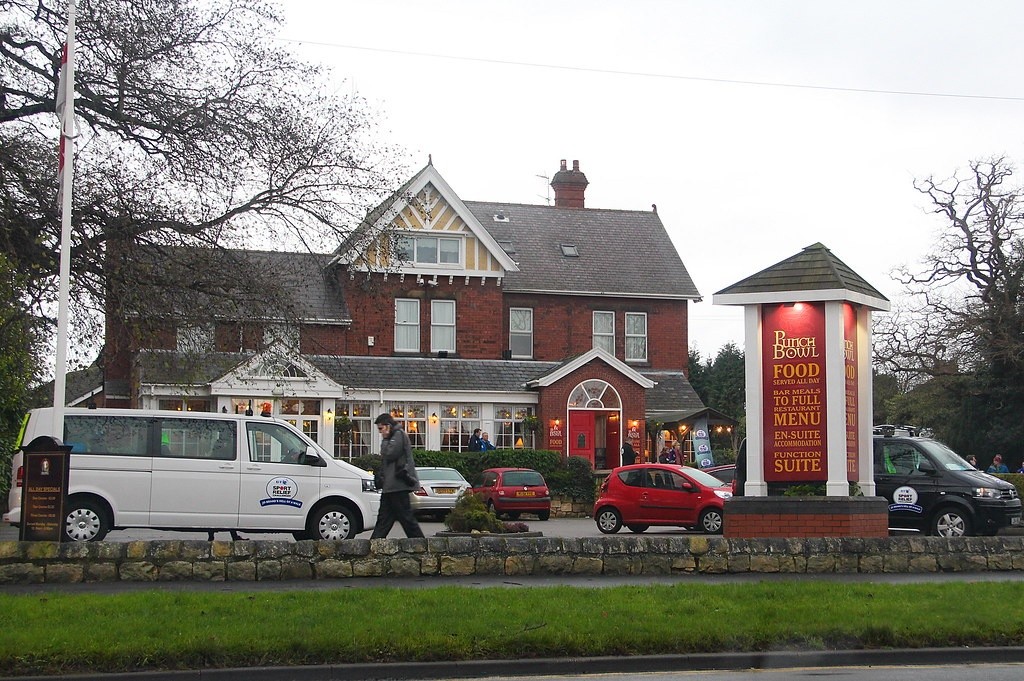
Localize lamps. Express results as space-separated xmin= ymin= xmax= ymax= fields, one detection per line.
xmin=555 ymin=417 xmax=560 ymax=426
xmin=327 ymin=409 xmax=332 ymax=420
xmin=432 ymin=413 xmax=437 ymax=423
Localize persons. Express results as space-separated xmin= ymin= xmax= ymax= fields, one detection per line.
xmin=659 ymin=446 xmax=670 ymax=464
xmin=986 ymin=454 xmax=1009 ymax=473
xmin=1016 ymin=461 xmax=1024 ymax=473
xmin=370 ymin=413 xmax=425 ymax=539
xmin=621 ymin=436 xmax=640 ymax=467
xmin=670 ymin=440 xmax=684 ymax=465
xmin=966 ymin=455 xmax=977 ymax=468
xmin=161 ymin=431 xmax=172 ymax=456
xmin=206 ymin=530 xmax=250 ymax=541
xmin=480 ymin=432 xmax=496 ymax=452
xmin=467 ymin=428 xmax=483 ymax=452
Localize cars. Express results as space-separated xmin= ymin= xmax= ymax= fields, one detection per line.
xmin=472 ymin=467 xmax=552 ymax=522
xmin=414 ymin=466 xmax=474 ymax=520
xmin=593 ymin=464 xmax=735 ymax=536
xmin=673 ymin=465 xmax=737 ymax=489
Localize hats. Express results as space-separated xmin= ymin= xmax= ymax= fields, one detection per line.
xmin=994 ymin=457 xmax=1000 ymax=463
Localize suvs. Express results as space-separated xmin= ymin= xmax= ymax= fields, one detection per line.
xmin=873 ymin=425 xmax=1023 ymax=535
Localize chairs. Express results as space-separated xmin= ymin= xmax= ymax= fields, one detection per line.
xmin=160 ymin=444 xmax=172 ymax=456
xmin=655 ymin=473 xmax=664 ymax=488
xmin=212 ymin=438 xmax=233 ymax=458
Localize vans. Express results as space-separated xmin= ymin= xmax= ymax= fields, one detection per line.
xmin=4 ymin=390 xmax=385 ymax=545
